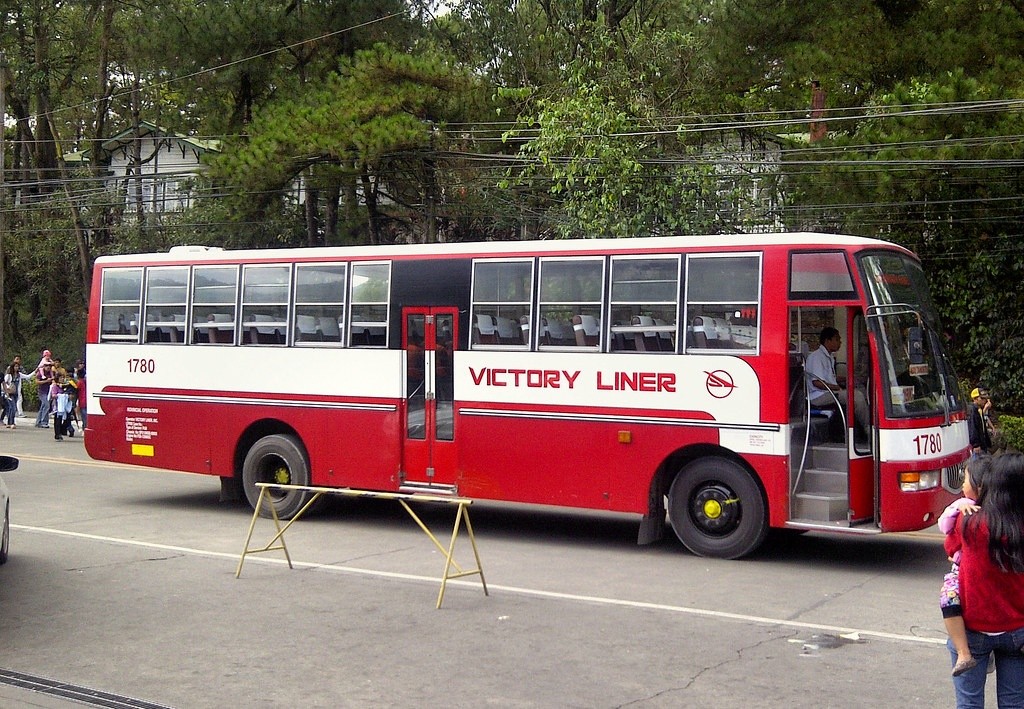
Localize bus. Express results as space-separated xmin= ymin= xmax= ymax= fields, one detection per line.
xmin=82 ymin=231 xmax=978 ymax=564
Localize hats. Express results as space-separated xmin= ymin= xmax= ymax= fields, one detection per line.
xmin=43 ymin=350 xmax=51 ymax=357
xmin=970 ymin=388 xmax=990 ymax=400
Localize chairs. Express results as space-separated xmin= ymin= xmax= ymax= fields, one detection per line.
xmin=102 ymin=306 xmax=808 ymax=398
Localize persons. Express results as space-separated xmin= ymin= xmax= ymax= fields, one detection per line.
xmin=0 ymin=362 xmax=39 ymax=429
xmin=35 ymin=350 xmax=86 ymax=440
xmin=944 ymin=452 xmax=1024 ymax=709
xmin=937 ymin=456 xmax=995 ymax=678
xmin=5 ymin=355 xmax=27 ymax=418
xmin=968 ymin=387 xmax=996 ymax=460
xmin=805 ymin=327 xmax=870 ymax=433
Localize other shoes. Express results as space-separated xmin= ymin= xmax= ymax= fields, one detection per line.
xmin=952 ymin=656 xmax=977 ymax=677
xmin=69 ymin=429 xmax=75 ymax=438
xmin=38 ymin=424 xmax=49 ymax=428
xmin=986 ymin=651 xmax=996 ymax=674
xmin=42 ymin=375 xmax=46 ymax=379
xmin=53 ymin=434 xmax=62 ymax=441
